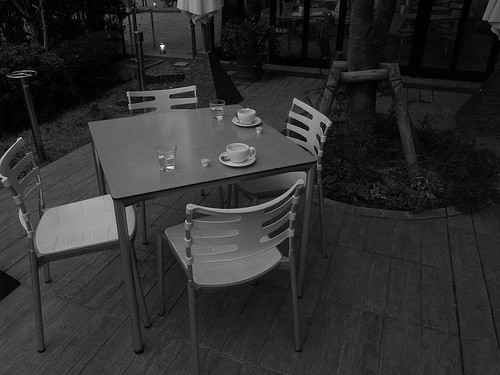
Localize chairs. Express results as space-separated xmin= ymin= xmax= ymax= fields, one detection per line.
xmin=231 ymin=98 xmax=332 ymax=297
xmin=157 ymin=178 xmax=304 ymax=375
xmin=390 ymin=8 xmax=461 ymax=67
xmin=127 ymin=85 xmax=225 ymax=245
xmin=0 ymin=137 xmax=151 ymax=353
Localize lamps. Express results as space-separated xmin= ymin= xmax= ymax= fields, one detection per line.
xmin=160 ymin=42 xmax=167 ymax=54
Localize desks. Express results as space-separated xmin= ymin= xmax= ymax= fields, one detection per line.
xmin=279 ymin=14 xmax=330 ymax=52
xmin=405 ymin=12 xmax=456 ymax=64
xmin=88 ymin=104 xmax=319 ymax=354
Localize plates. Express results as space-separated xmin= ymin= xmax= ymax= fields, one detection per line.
xmin=231 ymin=116 xmax=262 ymax=127
xmin=218 ymin=152 xmax=256 ymax=167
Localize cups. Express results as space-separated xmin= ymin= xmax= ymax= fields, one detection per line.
xmin=237 ymin=108 xmax=256 ymax=125
xmin=154 ymin=143 xmax=177 ymax=172
xmin=209 ymin=99 xmax=225 ymax=120
xmin=226 ymin=142 xmax=256 ymax=163
